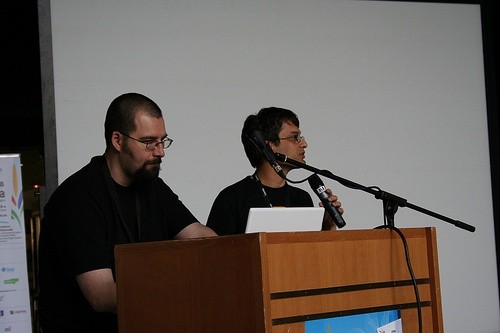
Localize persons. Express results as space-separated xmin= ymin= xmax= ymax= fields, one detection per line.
xmin=37 ymin=93 xmax=217 ymax=333
xmin=206 ymin=107 xmax=343 ymax=235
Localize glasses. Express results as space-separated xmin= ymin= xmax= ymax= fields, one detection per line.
xmin=279 ymin=135 xmax=302 ymax=143
xmin=112 ymin=128 xmax=173 ymax=150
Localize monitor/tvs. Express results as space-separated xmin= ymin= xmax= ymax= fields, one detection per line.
xmin=246 ymin=208 xmax=325 ymax=234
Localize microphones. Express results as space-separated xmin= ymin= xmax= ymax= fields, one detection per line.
xmin=246 ymin=127 xmax=286 ymax=180
xmin=308 ymin=174 xmax=346 ymax=228
